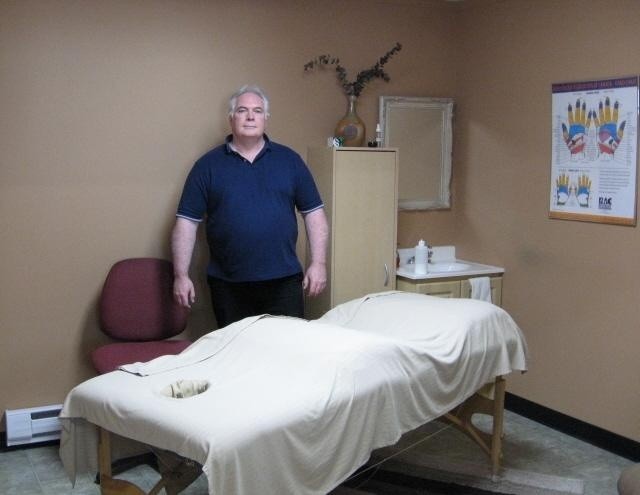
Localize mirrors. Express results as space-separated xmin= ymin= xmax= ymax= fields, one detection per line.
xmin=379 ymin=94 xmax=452 ymax=212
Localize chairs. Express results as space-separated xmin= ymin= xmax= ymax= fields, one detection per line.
xmin=94 ymin=256 xmax=192 ymax=482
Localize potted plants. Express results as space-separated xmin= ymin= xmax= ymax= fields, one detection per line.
xmin=302 ymin=39 xmax=402 ymax=148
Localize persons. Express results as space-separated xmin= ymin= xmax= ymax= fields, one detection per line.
xmin=168 ymin=85 xmax=330 ymax=328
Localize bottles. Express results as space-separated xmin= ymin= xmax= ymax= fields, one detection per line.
xmin=413 ymin=238 xmax=428 ymax=275
xmin=375 ymin=123 xmax=382 ymax=148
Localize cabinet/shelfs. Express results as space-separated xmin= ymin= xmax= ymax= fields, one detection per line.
xmin=395 ymin=258 xmax=506 ymax=301
xmin=304 ymin=146 xmax=398 ymax=313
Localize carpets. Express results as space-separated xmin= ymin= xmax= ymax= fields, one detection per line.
xmin=328 ymin=446 xmax=585 ymax=494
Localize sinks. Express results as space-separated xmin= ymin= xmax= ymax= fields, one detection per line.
xmin=396 ymin=259 xmax=504 ymax=280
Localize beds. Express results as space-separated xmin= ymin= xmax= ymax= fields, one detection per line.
xmin=57 ymin=288 xmax=528 ymax=494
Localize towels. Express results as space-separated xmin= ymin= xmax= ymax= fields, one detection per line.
xmin=469 ymin=277 xmax=491 ymax=302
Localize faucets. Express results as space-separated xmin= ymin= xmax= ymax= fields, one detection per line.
xmin=407 ymin=244 xmax=433 ymax=265
